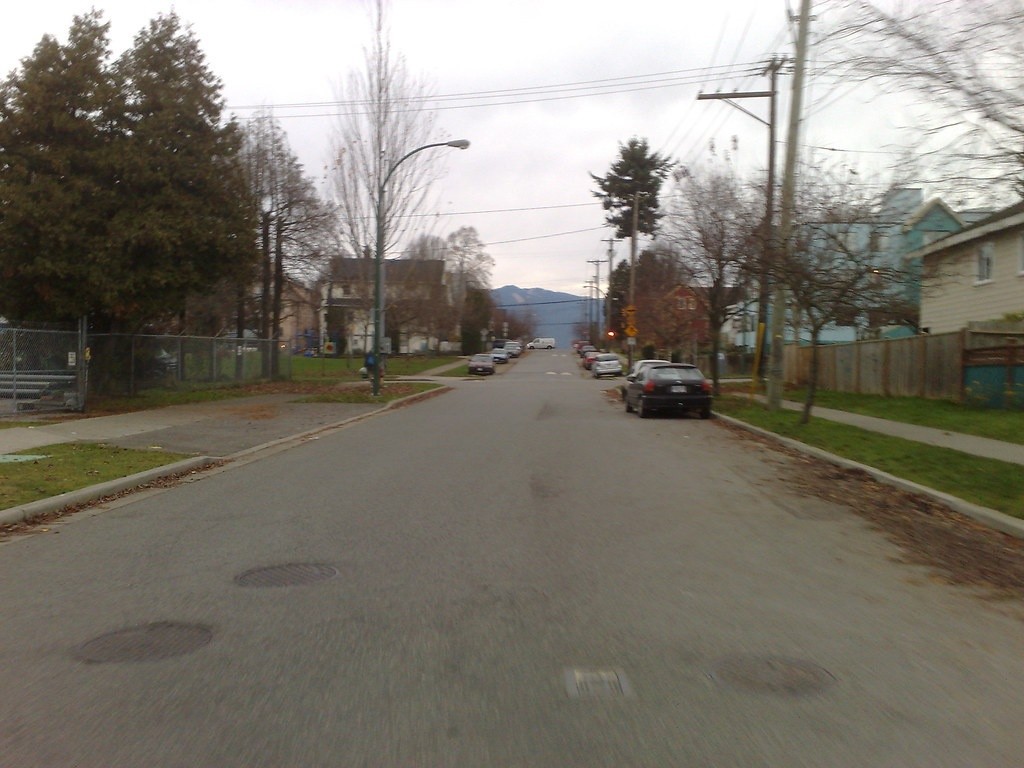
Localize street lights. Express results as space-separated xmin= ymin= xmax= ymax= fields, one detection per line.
xmin=583 ymin=285 xmax=610 ymax=354
xmin=370 ymin=138 xmax=471 ymax=397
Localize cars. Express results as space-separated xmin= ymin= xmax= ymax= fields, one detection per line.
xmin=141 ymin=347 xmax=177 ymax=383
xmin=579 ymin=346 xmax=596 ymax=359
xmin=623 ymin=363 xmax=713 ymax=419
xmin=591 ymin=354 xmax=622 ymax=379
xmin=633 ymin=360 xmax=680 ymax=380
xmin=468 ymin=354 xmax=496 ymax=376
xmin=576 ymin=340 xmax=591 ymax=354
xmin=490 ymin=348 xmax=509 ymax=363
xmin=582 ymin=352 xmax=601 ymax=370
xmin=492 ymin=338 xmax=523 ymax=358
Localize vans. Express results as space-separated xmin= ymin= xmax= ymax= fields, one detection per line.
xmin=526 ymin=337 xmax=555 ymax=350
